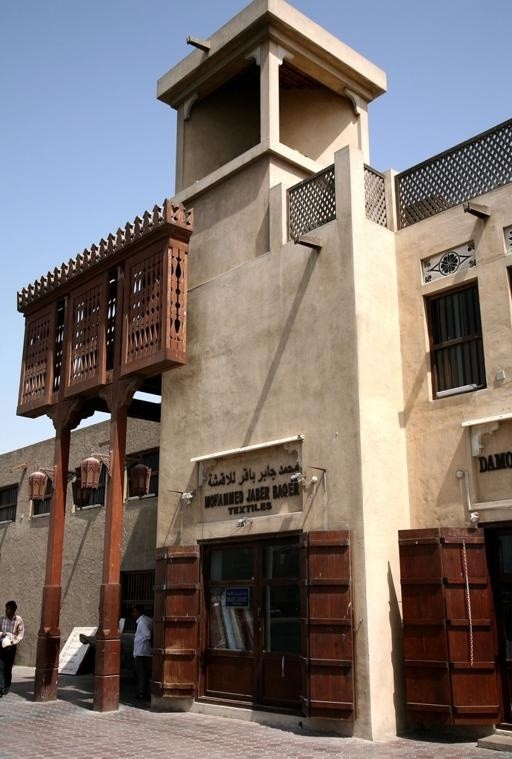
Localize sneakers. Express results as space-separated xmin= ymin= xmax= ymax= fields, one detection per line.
xmin=136 ymin=693 xmax=146 ymax=700
xmin=1 ymin=688 xmax=9 ymax=695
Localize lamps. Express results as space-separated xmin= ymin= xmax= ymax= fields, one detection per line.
xmin=182 ymin=493 xmax=194 ymax=505
xmin=237 ymin=517 xmax=252 ymax=527
xmin=291 ymin=473 xmax=317 ymax=487
xmin=470 ymin=512 xmax=479 ymax=523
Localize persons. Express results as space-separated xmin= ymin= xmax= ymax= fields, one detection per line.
xmin=0 ymin=599 xmax=25 ymax=698
xmin=130 ymin=601 xmax=154 ymax=702
